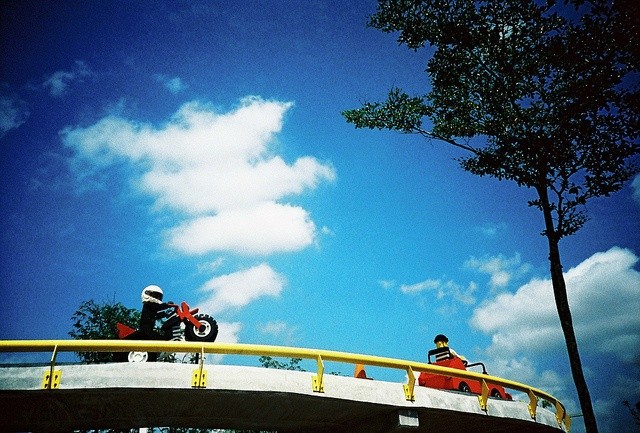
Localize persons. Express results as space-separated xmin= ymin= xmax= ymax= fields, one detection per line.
xmin=434 ymin=333 xmax=471 ymax=363
xmin=138 ymin=285 xmax=175 ymax=334
